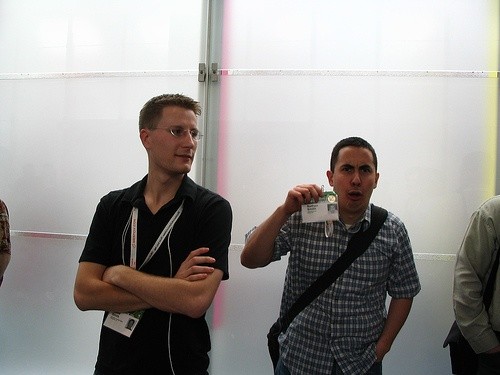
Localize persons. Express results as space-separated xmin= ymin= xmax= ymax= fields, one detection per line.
xmin=73 ymin=94 xmax=232 ymax=375
xmin=442 ymin=195 xmax=500 ymax=375
xmin=328 ymin=204 xmax=337 ymax=215
xmin=240 ymin=137 xmax=421 ymax=375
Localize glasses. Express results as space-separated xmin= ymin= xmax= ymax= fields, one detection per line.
xmin=152 ymin=127 xmax=201 ymax=142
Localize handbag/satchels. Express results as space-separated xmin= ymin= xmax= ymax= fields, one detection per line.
xmin=442 ymin=320 xmax=468 ymax=375
xmin=266 ymin=319 xmax=281 ymax=374
